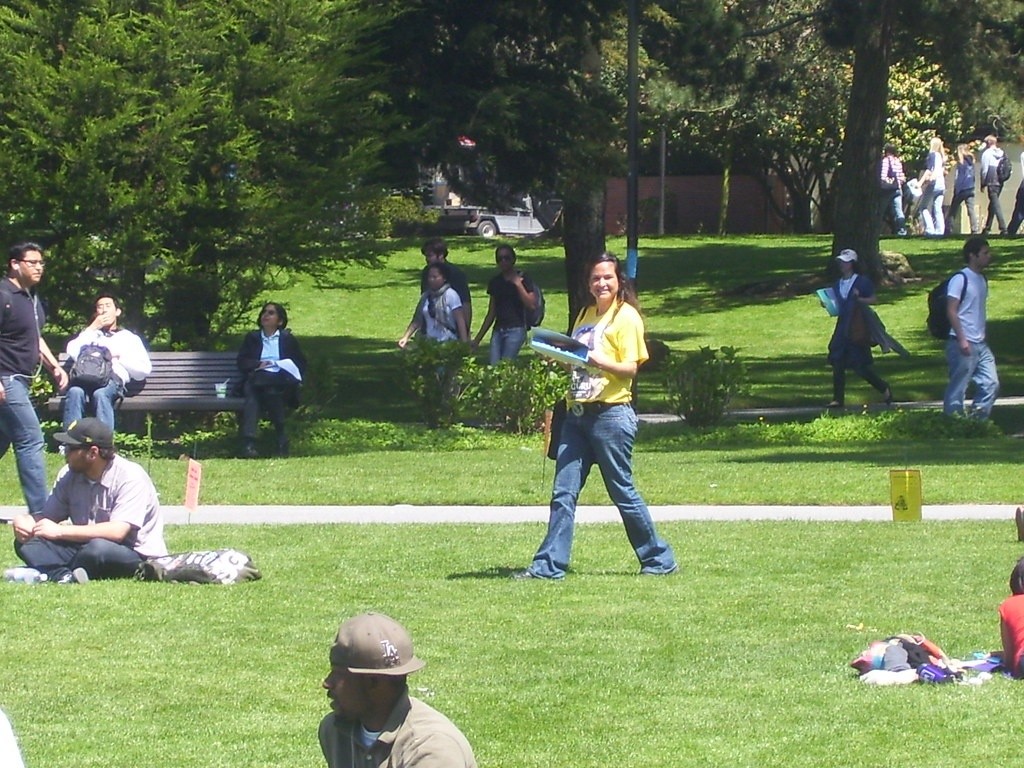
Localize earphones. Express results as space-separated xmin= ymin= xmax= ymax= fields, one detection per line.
xmin=15 ymin=264 xmax=20 ymax=269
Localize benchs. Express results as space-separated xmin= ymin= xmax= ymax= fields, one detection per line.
xmin=49 ymin=351 xmax=245 ymax=433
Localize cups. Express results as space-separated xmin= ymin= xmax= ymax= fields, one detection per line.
xmin=214 ymin=383 xmax=227 ymax=399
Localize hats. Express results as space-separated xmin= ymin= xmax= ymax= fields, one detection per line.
xmin=331 ymin=614 xmax=425 ymax=676
xmin=53 ymin=418 xmax=114 ymax=449
xmin=836 ymin=249 xmax=859 ymax=263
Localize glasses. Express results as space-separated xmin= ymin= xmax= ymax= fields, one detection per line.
xmin=495 ymin=253 xmax=514 ymax=263
xmin=260 ymin=308 xmax=278 ymax=315
xmin=16 ymin=256 xmax=45 ymax=266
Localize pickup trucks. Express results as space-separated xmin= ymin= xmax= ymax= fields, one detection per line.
xmin=437 ymin=193 xmax=544 ymax=237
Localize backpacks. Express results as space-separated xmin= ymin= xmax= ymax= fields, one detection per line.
xmin=998 ymin=151 xmax=1013 ymax=182
xmin=518 ymin=271 xmax=546 ymax=327
xmin=69 ymin=344 xmax=114 ymax=387
xmin=925 ymin=272 xmax=968 ymax=339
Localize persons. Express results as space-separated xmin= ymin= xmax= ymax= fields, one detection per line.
xmin=943 ymin=237 xmax=1000 ymax=420
xmin=0 ymin=241 xmax=69 ymax=521
xmin=879 ymin=143 xmax=907 ymax=236
xmin=944 ymin=144 xmax=979 ymax=236
xmin=915 ymin=137 xmax=945 ymax=235
xmin=318 ymin=612 xmax=478 ymax=768
xmin=821 ymin=249 xmax=892 ymax=409
xmin=980 ymin=135 xmax=1008 ymax=236
xmin=1007 ymin=151 xmax=1024 ymax=235
xmin=397 ymin=237 xmax=473 ymax=430
xmin=236 ymin=302 xmax=306 ymax=459
xmin=470 ymin=245 xmax=537 ymax=364
xmin=57 ymin=294 xmax=153 ymax=457
xmin=13 ymin=416 xmax=168 ymax=583
xmin=512 ymin=252 xmax=678 ymax=581
xmin=998 ymin=556 xmax=1024 ymax=678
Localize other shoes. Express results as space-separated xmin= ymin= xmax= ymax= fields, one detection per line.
xmin=826 ymin=400 xmax=845 ymax=407
xmin=241 ymin=439 xmax=260 ymax=459
xmin=53 ymin=568 xmax=89 ymax=585
xmin=882 ymin=383 xmax=893 ymax=404
xmin=274 ymin=441 xmax=291 ymax=457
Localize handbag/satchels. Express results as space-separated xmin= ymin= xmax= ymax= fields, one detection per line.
xmin=881 ymin=172 xmax=899 ymax=191
